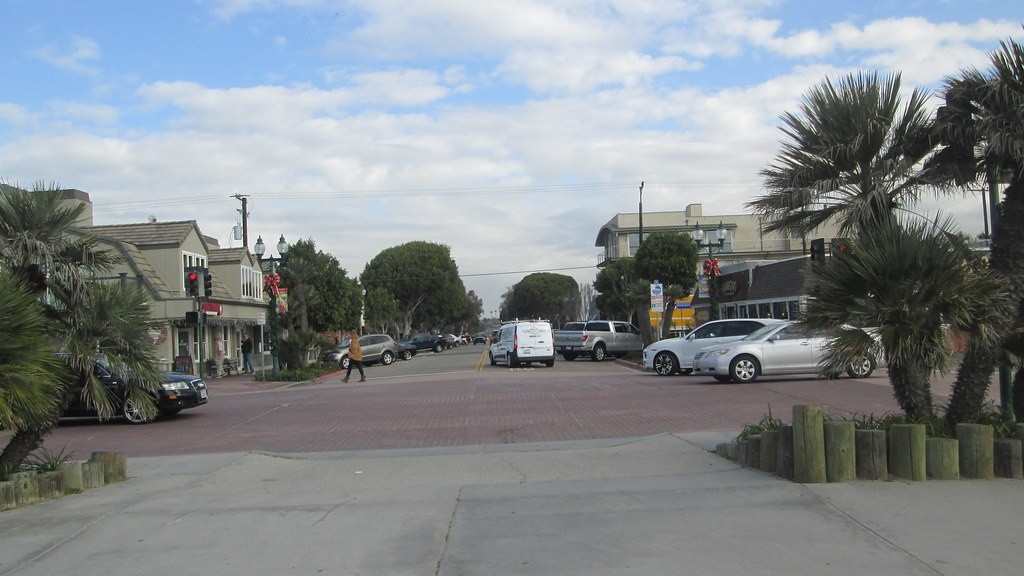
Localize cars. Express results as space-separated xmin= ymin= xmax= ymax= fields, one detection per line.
xmin=643 ymin=318 xmax=788 ymax=376
xmin=401 ymin=333 xmax=472 ymax=353
xmin=471 ymin=333 xmax=487 ymax=345
xmin=42 ymin=350 xmax=209 ymax=424
xmin=692 ymin=320 xmax=888 ymax=384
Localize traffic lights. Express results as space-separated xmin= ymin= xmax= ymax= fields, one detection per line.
xmin=831 ymin=238 xmax=853 ymax=257
xmin=203 ymin=267 xmax=212 ymax=298
xmin=188 ymin=266 xmax=199 ymax=296
xmin=810 ymin=238 xmax=826 ymax=272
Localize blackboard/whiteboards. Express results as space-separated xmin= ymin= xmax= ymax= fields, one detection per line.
xmin=174 ymin=355 xmax=194 ymax=374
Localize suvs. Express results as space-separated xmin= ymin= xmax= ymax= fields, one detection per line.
xmin=321 ymin=334 xmax=416 ymax=369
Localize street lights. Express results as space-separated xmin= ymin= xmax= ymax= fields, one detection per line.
xmin=255 ymin=234 xmax=288 ymax=375
xmin=692 ymin=220 xmax=728 ymax=321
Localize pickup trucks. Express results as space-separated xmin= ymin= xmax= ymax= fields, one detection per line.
xmin=552 ymin=320 xmax=645 ymax=361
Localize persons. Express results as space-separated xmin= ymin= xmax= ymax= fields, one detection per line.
xmin=241 ymin=333 xmax=255 ymax=375
xmin=340 ymin=330 xmax=366 ymax=383
xmin=214 ymin=336 xmax=227 ymax=378
xmin=335 ymin=338 xmax=338 ymax=344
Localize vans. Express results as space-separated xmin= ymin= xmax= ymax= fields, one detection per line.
xmin=489 ymin=318 xmax=555 ymax=368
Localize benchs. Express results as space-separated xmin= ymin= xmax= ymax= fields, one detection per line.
xmin=205 ymin=357 xmax=240 ymax=377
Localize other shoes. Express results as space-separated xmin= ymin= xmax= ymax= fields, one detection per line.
xmin=216 ymin=376 xmax=222 ymax=378
xmin=251 ymin=372 xmax=255 ymax=376
xmin=222 ymin=374 xmax=227 ymax=376
xmin=341 ymin=379 xmax=349 ymax=383
xmin=359 ymin=375 xmax=366 ymax=381
